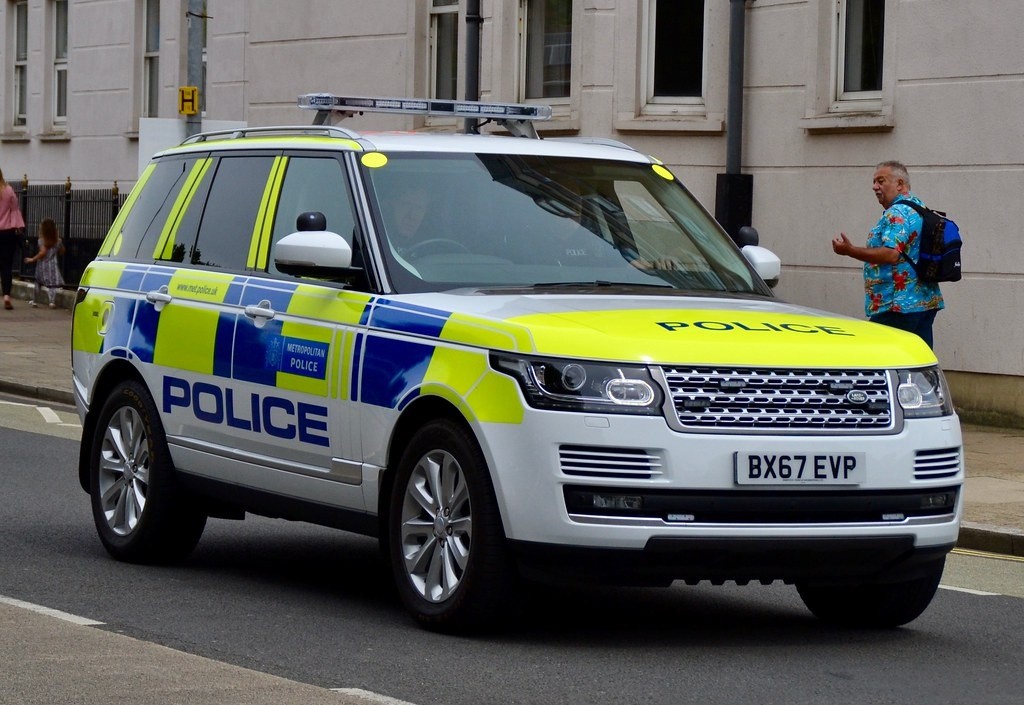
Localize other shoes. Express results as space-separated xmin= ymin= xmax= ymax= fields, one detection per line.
xmin=33 ymin=303 xmax=39 ymax=308
xmin=4 ymin=296 xmax=14 ymax=310
xmin=49 ymin=303 xmax=56 ymax=308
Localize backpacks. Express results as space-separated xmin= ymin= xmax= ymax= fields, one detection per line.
xmin=883 ymin=200 xmax=963 ymax=283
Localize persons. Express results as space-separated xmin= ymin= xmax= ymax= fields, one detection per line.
xmin=0 ymin=169 xmax=25 ymax=309
xmin=390 ymin=188 xmax=424 ymax=241
xmin=832 ymin=161 xmax=944 ymax=349
xmin=25 ymin=219 xmax=65 ymax=308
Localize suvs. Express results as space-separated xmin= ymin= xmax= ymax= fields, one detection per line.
xmin=69 ymin=94 xmax=965 ymax=629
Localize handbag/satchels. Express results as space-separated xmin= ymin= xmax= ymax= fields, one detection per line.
xmin=14 ymin=228 xmax=29 ymax=249
